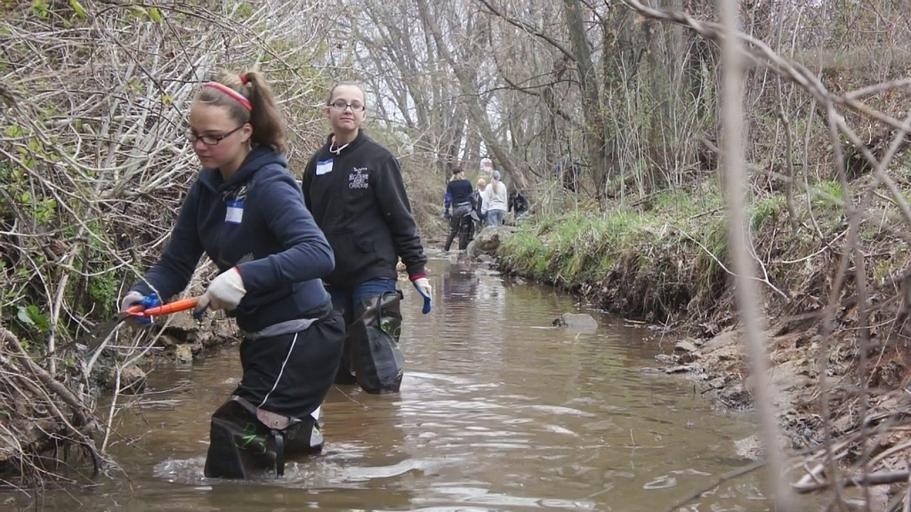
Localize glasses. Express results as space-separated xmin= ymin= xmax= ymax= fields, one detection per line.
xmin=185 ymin=126 xmax=243 ymax=145
xmin=328 ymin=102 xmax=365 ymax=111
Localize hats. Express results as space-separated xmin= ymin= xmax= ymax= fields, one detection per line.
xmin=453 ymin=167 xmax=464 ymax=174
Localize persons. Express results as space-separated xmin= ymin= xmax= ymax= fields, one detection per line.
xmin=301 ymin=83 xmax=432 ymax=396
xmin=120 ymin=70 xmax=350 ymax=479
xmin=480 ymin=170 xmax=509 ymax=228
xmin=472 ymin=178 xmax=528 ymax=231
xmin=442 ymin=166 xmax=476 ymax=250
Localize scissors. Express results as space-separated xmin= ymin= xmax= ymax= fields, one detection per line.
xmin=32 ymin=295 xmax=202 ymax=362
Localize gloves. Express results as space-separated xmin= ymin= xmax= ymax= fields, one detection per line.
xmin=194 ymin=267 xmax=245 ymax=317
xmin=121 ymin=290 xmax=160 ymax=329
xmin=412 ymin=278 xmax=432 ymax=315
